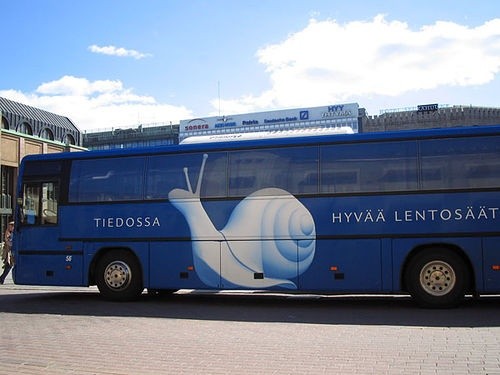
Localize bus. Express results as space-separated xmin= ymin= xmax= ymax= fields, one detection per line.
xmin=10 ymin=122 xmax=500 ymax=310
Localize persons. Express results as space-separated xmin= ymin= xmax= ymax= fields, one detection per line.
xmin=0 ymin=221 xmax=15 ymax=284
